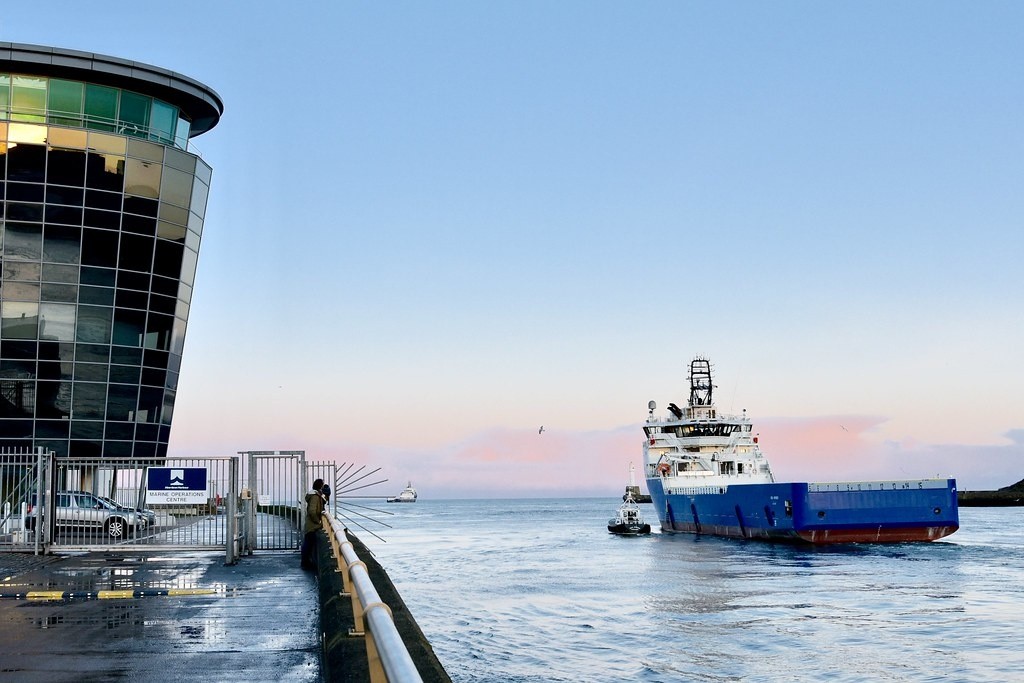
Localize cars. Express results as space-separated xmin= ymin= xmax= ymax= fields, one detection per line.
xmin=98 ymin=496 xmax=157 ymax=531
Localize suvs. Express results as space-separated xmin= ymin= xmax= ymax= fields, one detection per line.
xmin=25 ymin=489 xmax=143 ymax=539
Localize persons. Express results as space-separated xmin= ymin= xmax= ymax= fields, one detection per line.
xmin=301 ymin=479 xmax=331 ymax=569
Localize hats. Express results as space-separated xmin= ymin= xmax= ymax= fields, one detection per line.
xmin=322 ymin=484 xmax=330 ymax=496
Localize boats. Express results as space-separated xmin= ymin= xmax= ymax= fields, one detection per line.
xmin=623 ymin=462 xmax=655 ymax=503
xmin=643 ymin=359 xmax=960 ymax=546
xmin=386 ymin=480 xmax=417 ymax=503
xmin=607 ymin=487 xmax=651 ymax=535
